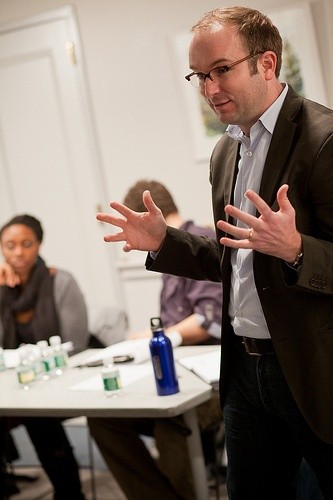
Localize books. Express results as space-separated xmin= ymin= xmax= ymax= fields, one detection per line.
xmin=78 ymin=332 xmax=182 ymax=367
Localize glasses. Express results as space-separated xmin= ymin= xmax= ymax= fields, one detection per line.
xmin=185 ymin=52 xmax=262 ymax=87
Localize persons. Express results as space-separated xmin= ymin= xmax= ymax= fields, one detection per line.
xmin=0 ymin=214 xmax=88 ymax=500
xmin=88 ymin=180 xmax=224 ymax=500
xmin=95 ymin=7 xmax=333 ymax=500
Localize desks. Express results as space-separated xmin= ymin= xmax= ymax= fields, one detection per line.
xmin=0 ymin=345 xmax=221 ymax=500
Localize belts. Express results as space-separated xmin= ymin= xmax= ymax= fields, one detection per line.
xmin=232 ymin=335 xmax=275 ymax=357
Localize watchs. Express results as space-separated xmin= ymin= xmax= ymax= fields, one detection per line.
xmin=292 ymin=252 xmax=303 ymax=267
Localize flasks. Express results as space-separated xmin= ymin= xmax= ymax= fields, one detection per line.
xmin=149 ymin=317 xmax=180 ymax=396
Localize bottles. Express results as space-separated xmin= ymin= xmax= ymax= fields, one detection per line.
xmin=49 ymin=336 xmax=70 ymax=375
xmin=15 ymin=345 xmax=38 ymax=392
xmin=35 ymin=340 xmax=56 ymax=381
xmin=100 ymin=352 xmax=124 ymax=398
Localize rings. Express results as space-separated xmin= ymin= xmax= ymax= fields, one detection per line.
xmin=249 ymin=228 xmax=253 ymax=239
